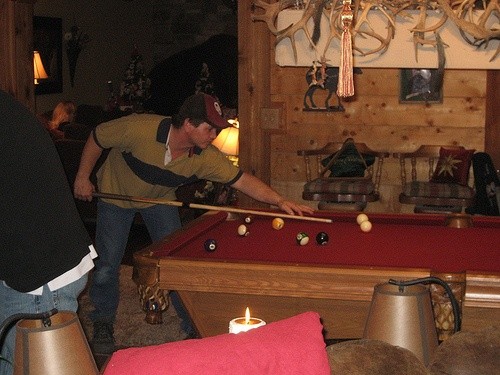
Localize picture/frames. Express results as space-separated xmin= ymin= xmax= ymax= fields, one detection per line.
xmin=398 ymin=68 xmax=443 ymax=105
xmin=33 ymin=16 xmax=64 ymax=95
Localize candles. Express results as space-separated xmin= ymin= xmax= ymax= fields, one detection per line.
xmin=228 ymin=307 xmax=267 ymax=335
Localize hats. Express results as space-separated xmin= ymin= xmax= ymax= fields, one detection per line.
xmin=182 ymin=94 xmax=234 ymax=129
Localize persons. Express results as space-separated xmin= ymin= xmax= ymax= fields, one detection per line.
xmin=0 ymin=90 xmax=99 ymax=375
xmin=73 ymin=93 xmax=316 ymax=358
xmin=49 ymin=101 xmax=94 ymax=141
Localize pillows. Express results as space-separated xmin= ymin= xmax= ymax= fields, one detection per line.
xmin=323 ymin=138 xmax=374 ymax=178
xmin=100 ymin=311 xmax=332 ymax=375
xmin=431 ymin=147 xmax=477 ymax=185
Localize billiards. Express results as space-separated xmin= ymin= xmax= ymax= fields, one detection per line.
xmin=356 ymin=214 xmax=368 ymax=225
xmin=242 ymin=214 xmax=254 ymax=224
xmin=204 ymin=239 xmax=217 ymax=253
xmin=316 ymin=232 xmax=329 ymax=245
xmin=296 ymin=231 xmax=309 ymax=246
xmin=360 ymin=221 xmax=373 ymax=232
xmin=272 ymin=217 xmax=284 ymax=230
xmin=237 ymin=224 xmax=250 ymax=237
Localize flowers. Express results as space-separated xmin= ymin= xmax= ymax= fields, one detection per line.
xmin=65 ymin=25 xmax=90 ymax=52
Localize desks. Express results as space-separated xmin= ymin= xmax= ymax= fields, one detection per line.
xmin=133 ymin=203 xmax=500 ymax=323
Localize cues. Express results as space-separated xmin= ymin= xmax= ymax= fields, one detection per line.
xmin=91 ymin=190 xmax=333 ymax=224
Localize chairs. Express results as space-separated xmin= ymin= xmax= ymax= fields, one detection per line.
xmin=297 ymin=142 xmax=389 ymax=212
xmin=393 ymin=144 xmax=483 ymax=213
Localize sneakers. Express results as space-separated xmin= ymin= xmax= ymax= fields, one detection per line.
xmin=92 ymin=326 xmax=114 ymax=356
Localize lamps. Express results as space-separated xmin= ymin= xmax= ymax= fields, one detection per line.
xmin=363 ymin=276 xmax=463 ymax=366
xmin=212 ymin=127 xmax=240 ymax=201
xmin=34 ymin=50 xmax=49 ymax=111
xmin=0 ymin=307 xmax=99 ymax=375
